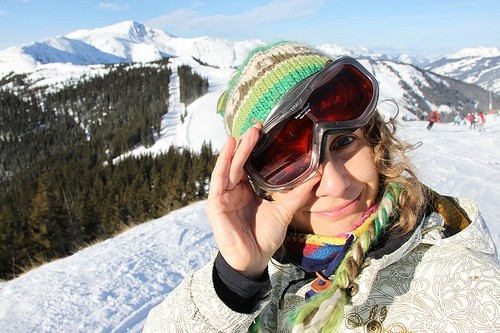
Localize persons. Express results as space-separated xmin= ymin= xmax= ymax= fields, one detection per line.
xmin=138 ymin=39 xmax=500 ymax=333
xmin=453 ymin=109 xmax=486 ymax=131
xmin=424 ymin=108 xmax=441 ymax=131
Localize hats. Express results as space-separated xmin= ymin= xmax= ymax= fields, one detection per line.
xmin=213 ymin=38 xmax=339 ymax=159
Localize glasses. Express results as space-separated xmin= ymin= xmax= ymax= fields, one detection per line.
xmin=239 ymin=54 xmax=383 ymax=193
